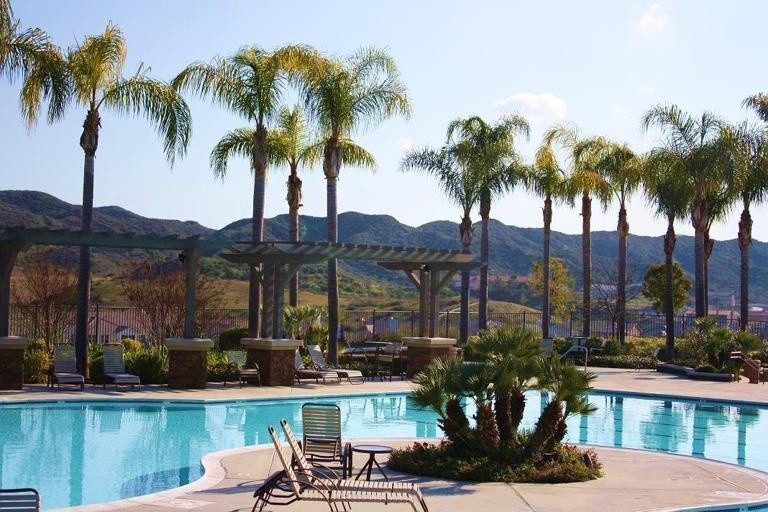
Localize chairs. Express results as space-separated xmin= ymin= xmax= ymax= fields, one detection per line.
xmin=535 ymin=336 xmax=590 ymax=370
xmin=222 ymin=350 xmax=264 ymax=387
xmin=46 ymin=342 xmax=86 ymax=392
xmin=294 ymin=342 xmax=406 ymax=384
xmin=265 ymin=403 xmax=428 ymax=510
xmin=100 ymin=343 xmax=141 ymax=391
